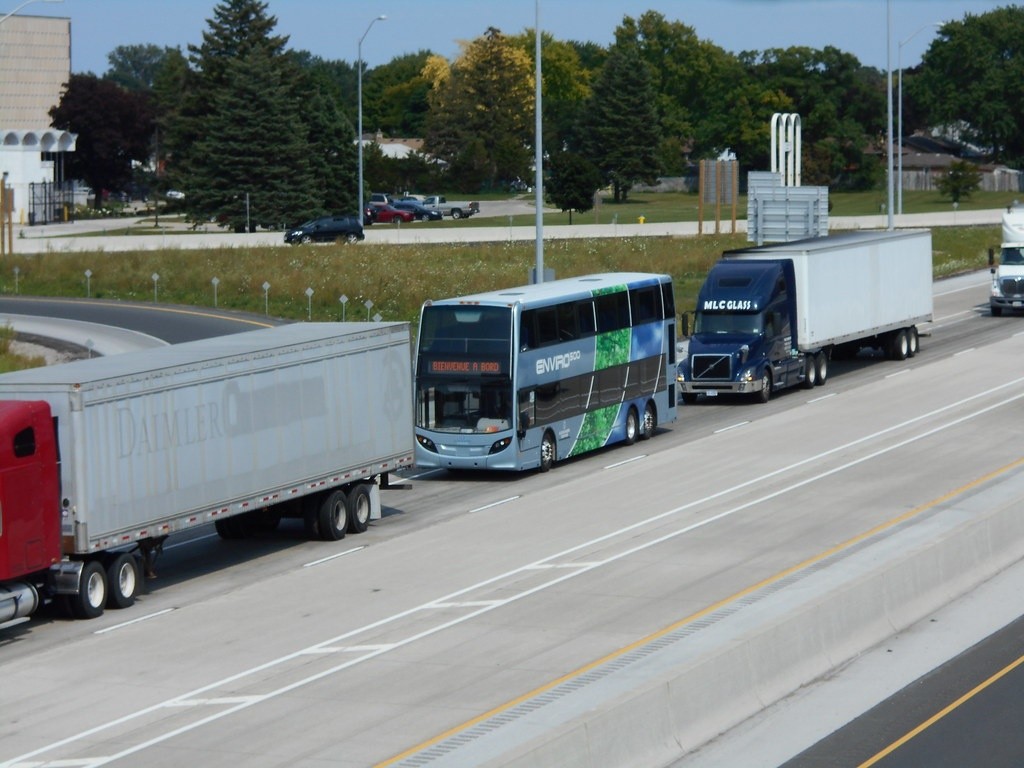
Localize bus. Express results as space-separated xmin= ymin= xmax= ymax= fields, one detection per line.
xmin=413 ymin=273 xmax=677 ymax=471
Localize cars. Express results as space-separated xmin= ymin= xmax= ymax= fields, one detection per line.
xmin=284 ymin=216 xmax=365 ymax=247
xmin=393 ymin=202 xmax=444 ymax=223
xmin=395 ymin=196 xmax=424 ymax=206
xmin=370 ymin=203 xmax=415 ymax=225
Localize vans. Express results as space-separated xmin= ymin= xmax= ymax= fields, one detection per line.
xmin=371 ymin=193 xmax=391 ymax=204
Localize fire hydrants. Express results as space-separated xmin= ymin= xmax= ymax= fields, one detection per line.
xmin=637 ymin=215 xmax=646 ymax=224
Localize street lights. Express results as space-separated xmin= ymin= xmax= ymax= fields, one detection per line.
xmin=358 ymin=15 xmax=388 ymax=225
xmin=898 ymin=23 xmax=944 ymax=216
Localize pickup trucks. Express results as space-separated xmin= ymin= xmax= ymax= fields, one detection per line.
xmin=421 ymin=197 xmax=480 ymax=220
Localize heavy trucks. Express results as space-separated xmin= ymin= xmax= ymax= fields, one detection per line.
xmin=987 ymin=212 xmax=1024 ymax=317
xmin=0 ymin=320 xmax=420 ymax=632
xmin=675 ymin=229 xmax=934 ymax=404
